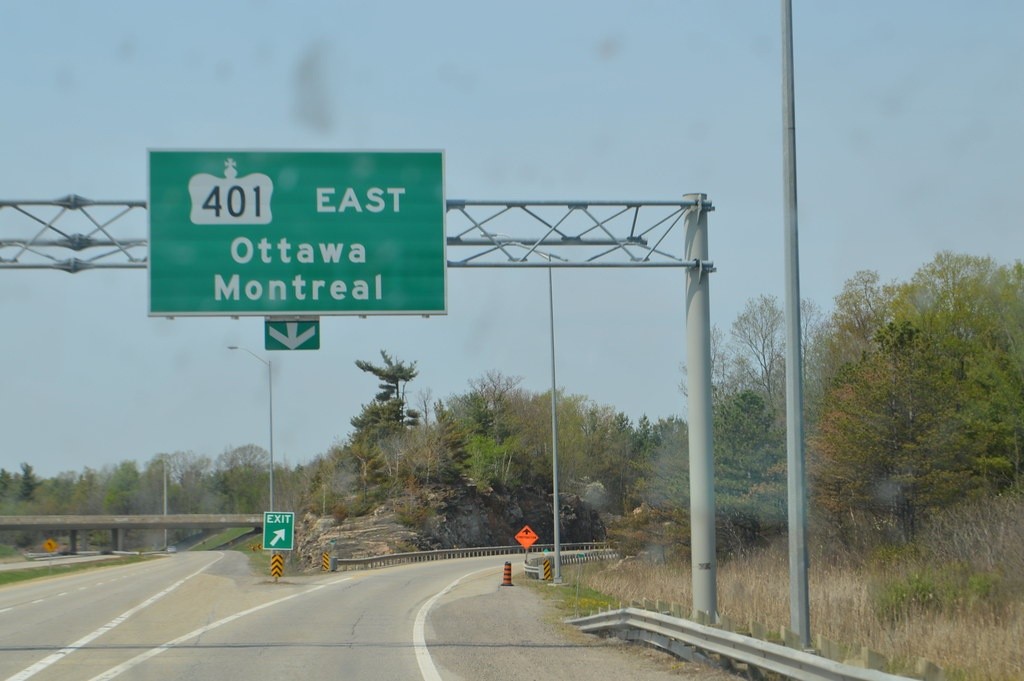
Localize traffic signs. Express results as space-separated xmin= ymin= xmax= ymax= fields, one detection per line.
xmin=142 ymin=149 xmax=448 ymax=318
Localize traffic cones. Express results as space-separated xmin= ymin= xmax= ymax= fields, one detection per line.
xmin=500 ymin=561 xmax=514 ymax=587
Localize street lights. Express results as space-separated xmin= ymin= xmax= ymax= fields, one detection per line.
xmin=479 ymin=231 xmax=561 ymax=584
xmin=227 ymin=344 xmax=274 ymax=516
